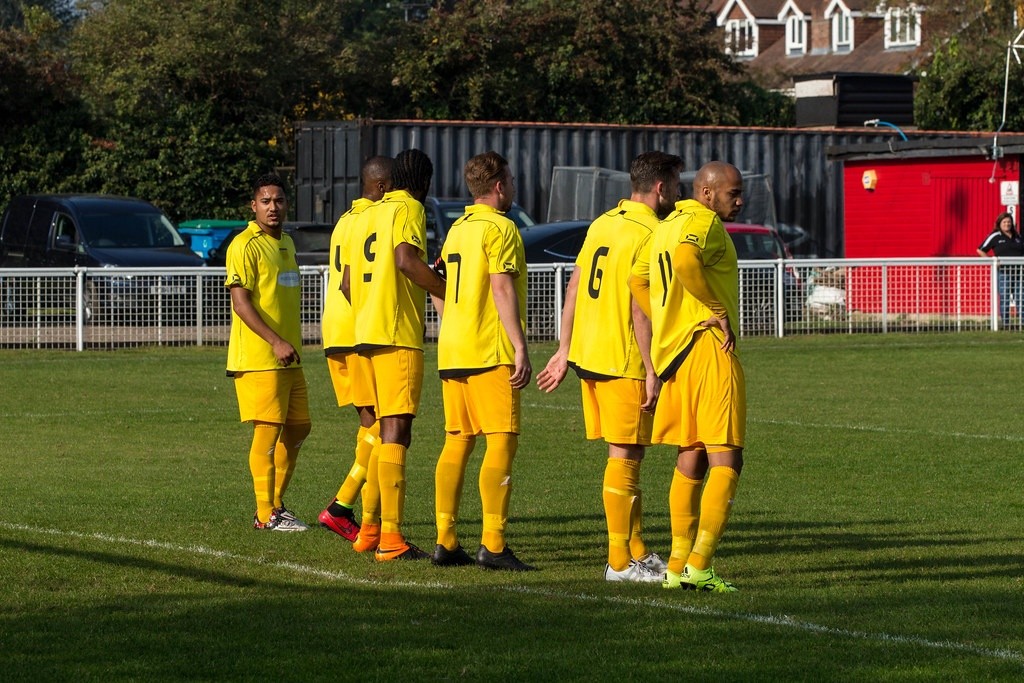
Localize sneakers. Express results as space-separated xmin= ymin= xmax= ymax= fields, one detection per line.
xmin=660 ymin=568 xmax=685 ymax=590
xmin=425 ymin=541 xmax=478 ymax=569
xmin=253 ymin=510 xmax=313 ymax=535
xmin=319 ymin=499 xmax=357 ymax=543
xmin=373 ymin=541 xmax=430 ymax=563
xmin=638 ymin=553 xmax=666 ymax=576
xmin=680 ymin=562 xmax=739 ymax=594
xmin=475 ymin=543 xmax=538 ymax=573
xmin=603 ymin=558 xmax=664 ymax=585
xmin=349 ymin=534 xmax=379 ymax=554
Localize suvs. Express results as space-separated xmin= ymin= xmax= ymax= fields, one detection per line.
xmin=0 ymin=192 xmax=208 ymax=324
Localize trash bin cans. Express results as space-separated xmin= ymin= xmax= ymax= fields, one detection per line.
xmin=178 ymin=219 xmax=248 ymax=259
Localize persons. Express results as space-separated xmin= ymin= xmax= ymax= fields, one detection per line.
xmin=534 ymin=152 xmax=686 ymax=582
xmin=225 ymin=176 xmax=312 ymax=531
xmin=319 ymin=157 xmax=395 ymax=541
xmin=431 ymin=152 xmax=535 ymax=570
xmin=353 ymin=149 xmax=448 ymax=558
xmin=629 ymin=162 xmax=745 ymax=594
xmin=977 ymin=213 xmax=1024 ymax=329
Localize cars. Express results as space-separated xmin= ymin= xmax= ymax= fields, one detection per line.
xmin=205 ymin=201 xmax=799 ymax=340
